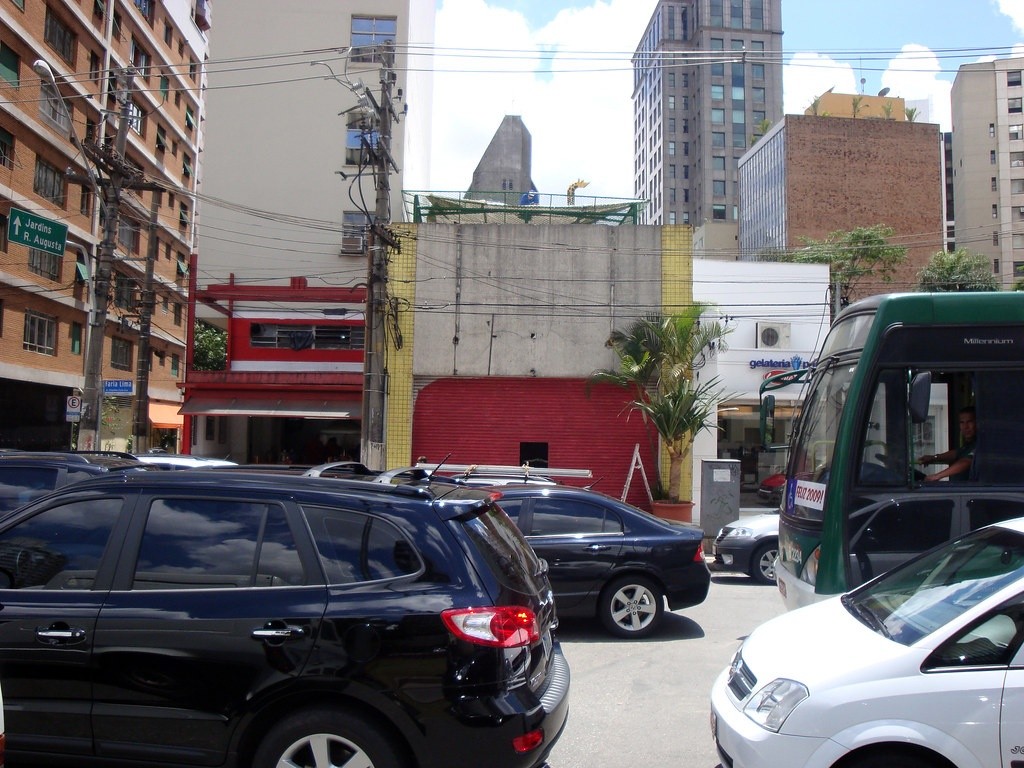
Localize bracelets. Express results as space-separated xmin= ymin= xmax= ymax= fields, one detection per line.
xmin=935 ymin=454 xmax=940 ymax=462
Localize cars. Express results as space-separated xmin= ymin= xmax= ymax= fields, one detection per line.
xmin=0 ymin=448 xmax=589 ymax=532
xmin=466 ymin=483 xmax=713 ymax=640
xmin=845 ymin=487 xmax=1024 ymax=589
xmin=712 ymin=513 xmax=781 ymax=586
xmin=758 ymin=470 xmax=786 ymax=508
xmin=709 ymin=517 xmax=1024 ymax=768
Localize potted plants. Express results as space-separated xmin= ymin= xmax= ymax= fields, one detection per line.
xmin=587 ymin=298 xmax=731 ymax=522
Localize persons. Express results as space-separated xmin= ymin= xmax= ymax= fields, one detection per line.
xmin=522 ymin=461 xmax=531 ymax=484
xmin=918 ymin=406 xmax=977 ymax=482
xmin=417 ymin=457 xmax=432 ymax=476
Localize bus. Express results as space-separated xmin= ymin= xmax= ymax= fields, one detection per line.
xmin=759 ymin=288 xmax=1024 ymax=613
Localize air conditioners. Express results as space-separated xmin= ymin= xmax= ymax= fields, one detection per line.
xmin=756 ymin=321 xmax=791 ymax=349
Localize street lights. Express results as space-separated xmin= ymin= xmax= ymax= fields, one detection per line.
xmin=32 ymin=58 xmax=118 ymax=451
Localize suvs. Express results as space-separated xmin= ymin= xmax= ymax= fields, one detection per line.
xmin=2 ymin=458 xmax=572 ymax=768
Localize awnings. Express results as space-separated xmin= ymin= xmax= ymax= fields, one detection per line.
xmin=178 ymin=398 xmax=365 ymax=420
